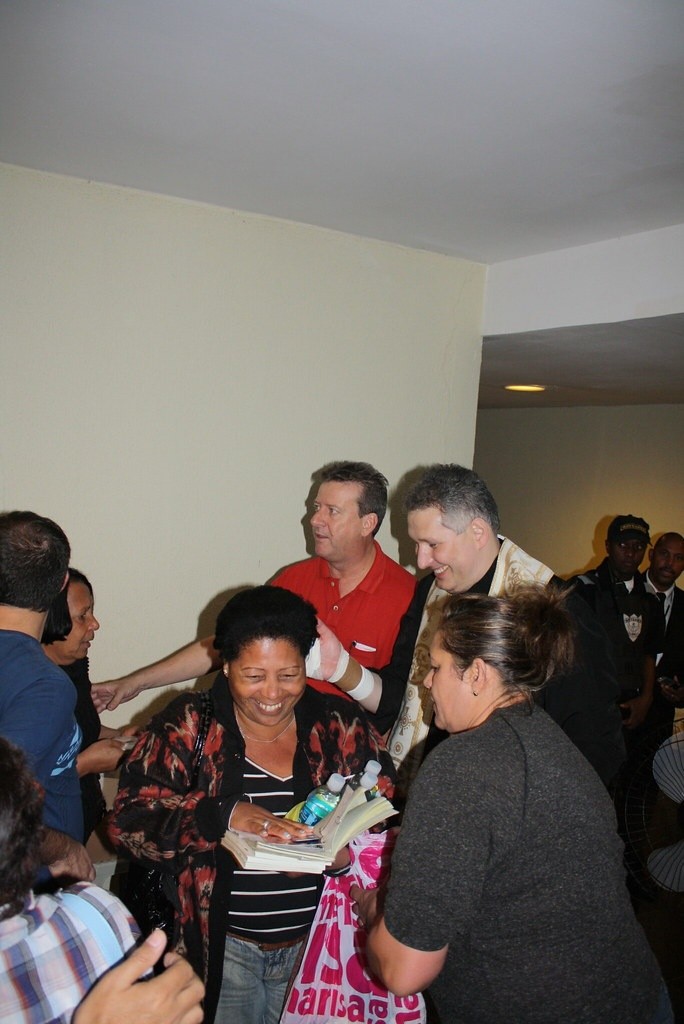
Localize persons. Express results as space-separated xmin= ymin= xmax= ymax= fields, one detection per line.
xmin=0 ymin=511 xmax=206 ymax=1024
xmin=307 ymin=465 xmax=684 ymax=1024
xmin=91 ymin=458 xmax=416 ymax=714
xmin=105 ymin=583 xmax=404 ymax=1024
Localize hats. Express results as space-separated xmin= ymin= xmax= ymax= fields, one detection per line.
xmin=608 ymin=514 xmax=653 ymax=546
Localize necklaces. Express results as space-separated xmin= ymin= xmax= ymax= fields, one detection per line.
xmin=241 ymin=716 xmax=295 ymax=742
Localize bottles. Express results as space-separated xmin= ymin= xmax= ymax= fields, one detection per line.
xmin=298 ymin=760 xmax=382 ymax=827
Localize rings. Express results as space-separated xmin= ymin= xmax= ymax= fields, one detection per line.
xmin=264 ymin=820 xmax=270 ymax=829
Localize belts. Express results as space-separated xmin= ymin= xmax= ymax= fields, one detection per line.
xmin=228 ymin=933 xmax=306 ymax=951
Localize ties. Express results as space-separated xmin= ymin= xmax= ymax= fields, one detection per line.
xmin=657 ymin=593 xmax=666 ymax=606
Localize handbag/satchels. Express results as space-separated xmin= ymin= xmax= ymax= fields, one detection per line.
xmin=278 ymin=829 xmax=427 ymax=1024
xmin=109 ymin=690 xmax=213 ymax=976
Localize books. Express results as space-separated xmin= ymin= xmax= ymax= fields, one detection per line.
xmin=220 ymin=786 xmax=398 ymax=875
